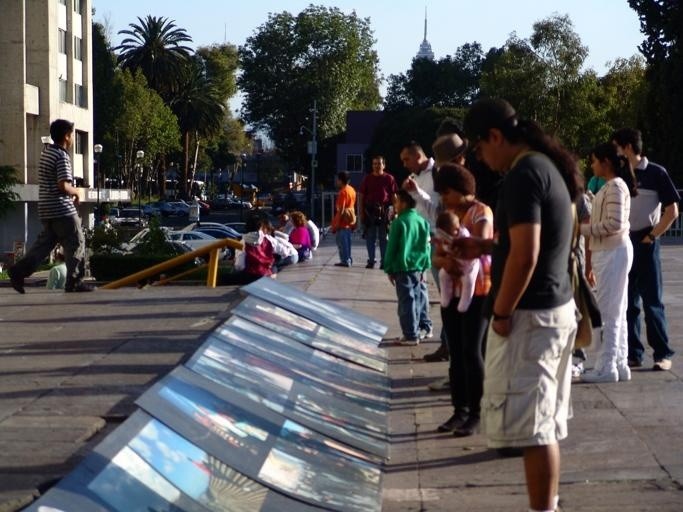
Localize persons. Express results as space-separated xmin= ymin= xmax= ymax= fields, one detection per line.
xmin=250 ymin=191 xmax=297 ymax=214
xmin=7 ymin=120 xmax=95 ymax=294
xmin=188 ymin=456 xmax=253 ymax=494
xmin=46 ymin=247 xmax=68 ymax=290
xmin=183 ymin=189 xmax=211 ymax=228
xmin=233 ymin=211 xmax=319 ymax=285
xmin=331 ymin=171 xmax=356 ymax=266
xmin=385 ymin=133 xmax=501 ymax=435
xmin=358 ymin=156 xmax=399 ymax=269
xmin=569 ymin=127 xmax=680 ymax=382
xmin=448 ymin=98 xmax=581 ymax=511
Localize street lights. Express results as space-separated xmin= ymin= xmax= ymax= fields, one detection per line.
xmin=239 ymin=152 xmax=248 ymax=205
xmin=138 ymin=151 xmax=147 ymax=216
xmin=300 ymin=126 xmax=320 ymax=218
xmin=95 ymin=144 xmax=104 ymax=210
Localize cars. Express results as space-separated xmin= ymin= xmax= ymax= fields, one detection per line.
xmin=108 ymin=189 xmax=288 ymax=262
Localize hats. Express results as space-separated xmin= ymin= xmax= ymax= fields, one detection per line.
xmin=431 ymin=133 xmax=468 ymax=168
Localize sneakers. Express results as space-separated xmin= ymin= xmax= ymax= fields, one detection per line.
xmin=572 ymin=357 xmax=672 ymax=382
xmin=7 ymin=263 xmax=24 ymax=293
xmin=334 ymin=262 xmax=374 ymax=268
xmin=64 ymin=281 xmax=98 ymax=291
xmin=427 ymin=377 xmax=480 ymax=436
xmin=393 ymin=326 xmax=449 ymax=362
